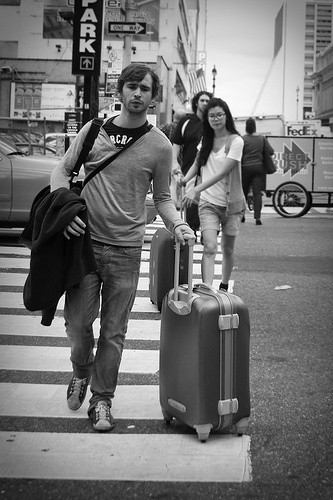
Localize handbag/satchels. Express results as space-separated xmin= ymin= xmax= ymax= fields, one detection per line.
xmin=262 ymin=136 xmax=276 ymax=173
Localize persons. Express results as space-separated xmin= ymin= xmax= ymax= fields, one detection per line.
xmin=180 ymin=97 xmax=248 ymax=292
xmin=50 ymin=62 xmax=196 ymax=431
xmin=241 ymin=116 xmax=274 ymax=225
xmin=171 ymin=90 xmax=213 ymax=245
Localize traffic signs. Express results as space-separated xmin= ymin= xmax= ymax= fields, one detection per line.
xmin=107 ymin=21 xmax=147 ymax=34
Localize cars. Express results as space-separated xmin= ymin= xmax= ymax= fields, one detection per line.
xmin=0 ymin=128 xmax=86 ymax=228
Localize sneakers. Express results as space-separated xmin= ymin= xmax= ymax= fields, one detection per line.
xmin=90 ymin=400 xmax=114 ymax=430
xmin=66 ymin=375 xmax=90 ymax=410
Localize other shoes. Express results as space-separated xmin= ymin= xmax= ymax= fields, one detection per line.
xmin=256 ymin=219 xmax=261 ymax=225
xmin=241 ymin=216 xmax=245 ymax=222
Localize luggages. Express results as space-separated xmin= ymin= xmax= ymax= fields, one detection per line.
xmin=148 ymin=181 xmax=188 ymax=313
xmin=160 ymin=233 xmax=250 ymax=442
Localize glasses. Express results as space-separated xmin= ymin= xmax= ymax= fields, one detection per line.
xmin=209 ymin=112 xmax=226 ymax=120
